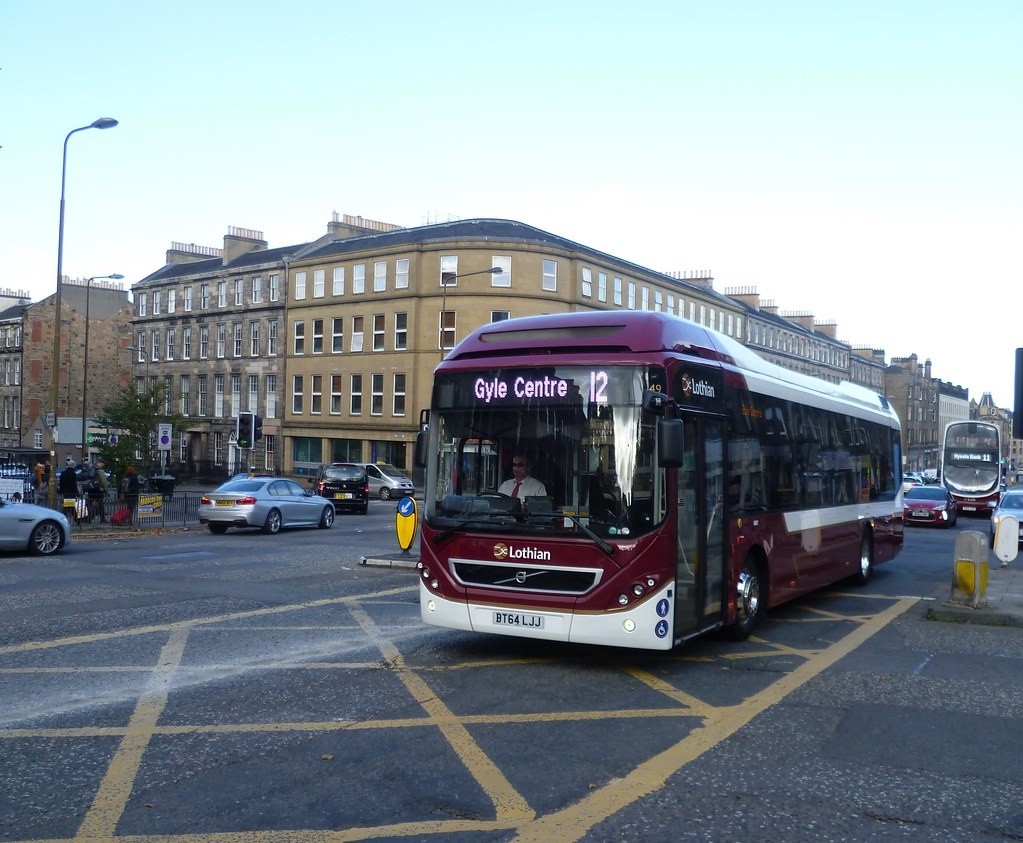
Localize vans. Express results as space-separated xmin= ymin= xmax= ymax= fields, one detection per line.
xmin=355 ymin=464 xmax=415 ymax=501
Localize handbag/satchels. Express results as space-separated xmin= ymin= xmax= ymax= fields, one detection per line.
xmin=75 ymin=497 xmax=89 ymax=518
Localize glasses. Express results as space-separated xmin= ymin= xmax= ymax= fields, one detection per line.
xmin=513 ymin=463 xmax=523 ymax=467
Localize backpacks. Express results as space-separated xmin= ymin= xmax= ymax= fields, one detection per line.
xmin=121 ymin=477 xmax=133 ymax=493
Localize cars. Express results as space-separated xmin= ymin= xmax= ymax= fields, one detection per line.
xmin=901 ymin=468 xmax=941 ymax=493
xmin=987 ymin=491 xmax=1023 ymax=549
xmin=0 ymin=463 xmax=30 ymax=477
xmin=196 ymin=478 xmax=336 ymax=535
xmin=0 ymin=496 xmax=72 ymax=557
xmin=902 ymin=486 xmax=957 ymax=531
xmin=308 ymin=463 xmax=369 ymax=516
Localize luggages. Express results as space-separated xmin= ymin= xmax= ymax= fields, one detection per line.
xmin=112 ymin=508 xmax=132 ymax=526
xmin=80 ymin=494 xmax=110 ymax=524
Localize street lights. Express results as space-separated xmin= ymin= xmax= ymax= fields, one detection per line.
xmin=47 ymin=118 xmax=120 ymax=510
xmin=441 ymin=267 xmax=503 ymax=362
xmin=126 ymin=347 xmax=148 ymax=397
xmin=81 ymin=275 xmax=126 ymax=465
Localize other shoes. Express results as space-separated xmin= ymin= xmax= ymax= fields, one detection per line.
xmin=99 ymin=518 xmax=110 ymax=524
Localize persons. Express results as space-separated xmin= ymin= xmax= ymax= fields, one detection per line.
xmin=85 ymin=462 xmax=110 ymax=524
xmin=60 ymin=457 xmax=81 ymax=525
xmin=497 ymin=454 xmax=547 ymax=504
xmin=29 ymin=461 xmax=52 ymax=506
xmin=118 ymin=465 xmax=140 ymax=525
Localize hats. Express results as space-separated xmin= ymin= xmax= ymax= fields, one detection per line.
xmin=66 ymin=461 xmax=76 ymax=469
xmin=97 ymin=458 xmax=105 ymax=464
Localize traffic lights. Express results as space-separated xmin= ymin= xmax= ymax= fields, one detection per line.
xmin=254 ymin=414 xmax=262 ymax=442
xmin=237 ymin=412 xmax=254 ymax=450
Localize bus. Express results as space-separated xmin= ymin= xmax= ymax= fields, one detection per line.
xmin=939 ymin=422 xmax=1002 ymax=515
xmin=414 ymin=309 xmax=905 ymax=650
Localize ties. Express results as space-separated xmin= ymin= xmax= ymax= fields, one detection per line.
xmin=511 ymin=482 xmax=523 ymax=499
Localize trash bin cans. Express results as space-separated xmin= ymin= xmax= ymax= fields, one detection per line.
xmin=149 ymin=476 xmax=175 ymax=493
